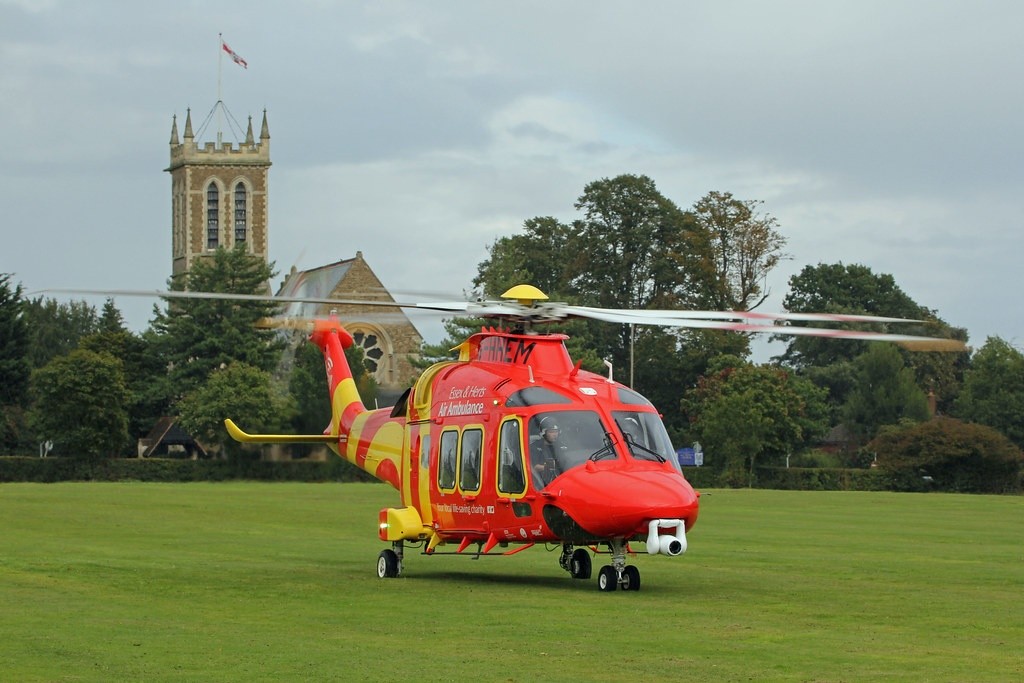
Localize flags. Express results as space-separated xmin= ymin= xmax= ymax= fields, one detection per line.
xmin=220 ymin=38 xmax=247 ymax=70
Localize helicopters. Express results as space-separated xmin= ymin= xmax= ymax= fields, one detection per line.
xmin=23 ymin=284 xmax=972 ymax=592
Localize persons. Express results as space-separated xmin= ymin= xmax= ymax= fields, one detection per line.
xmin=619 ymin=417 xmax=648 ymax=452
xmin=528 ymin=415 xmax=577 ymax=488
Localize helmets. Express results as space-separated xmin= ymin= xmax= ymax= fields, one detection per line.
xmin=540 ymin=416 xmax=559 ymax=432
xmin=622 ymin=418 xmax=639 ymax=434
